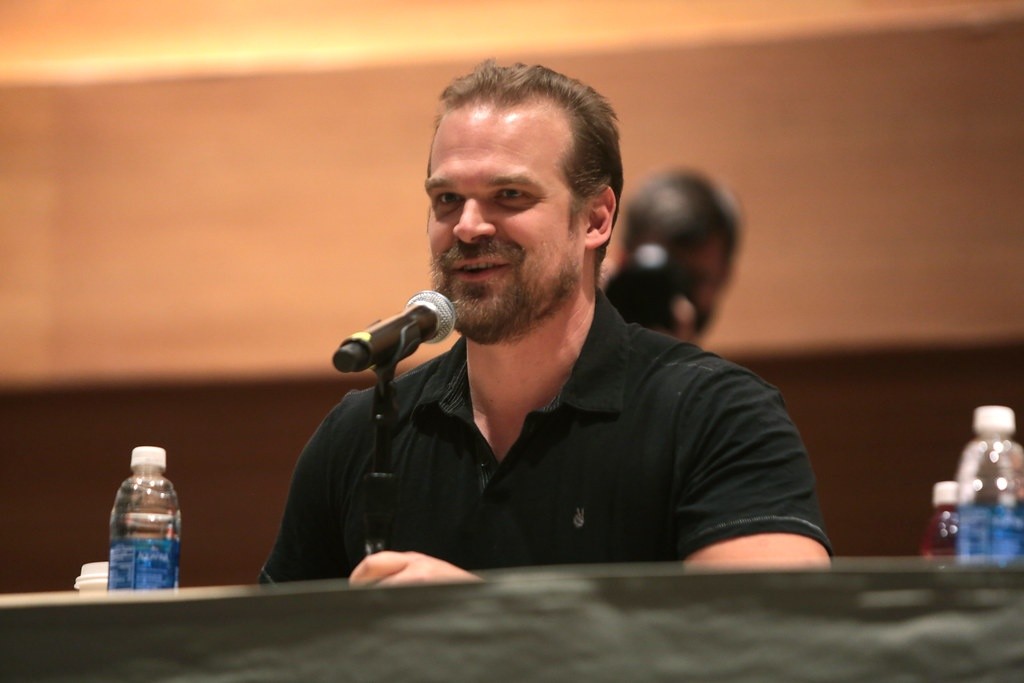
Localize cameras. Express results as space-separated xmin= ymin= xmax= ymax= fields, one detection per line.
xmin=603 ymin=263 xmax=691 ymax=334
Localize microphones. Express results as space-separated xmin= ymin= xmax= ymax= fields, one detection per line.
xmin=331 ymin=291 xmax=455 ymax=373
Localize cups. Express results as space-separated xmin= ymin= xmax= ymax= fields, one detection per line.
xmin=74 ymin=562 xmax=108 ymax=595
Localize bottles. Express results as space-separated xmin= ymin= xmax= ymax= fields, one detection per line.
xmin=923 ymin=481 xmax=957 ymax=557
xmin=957 ymin=405 xmax=1024 ymax=556
xmin=108 ymin=446 xmax=181 ymax=589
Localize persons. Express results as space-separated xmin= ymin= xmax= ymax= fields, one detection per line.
xmin=257 ymin=63 xmax=831 ymax=586
xmin=605 ymin=166 xmax=745 ymax=346
